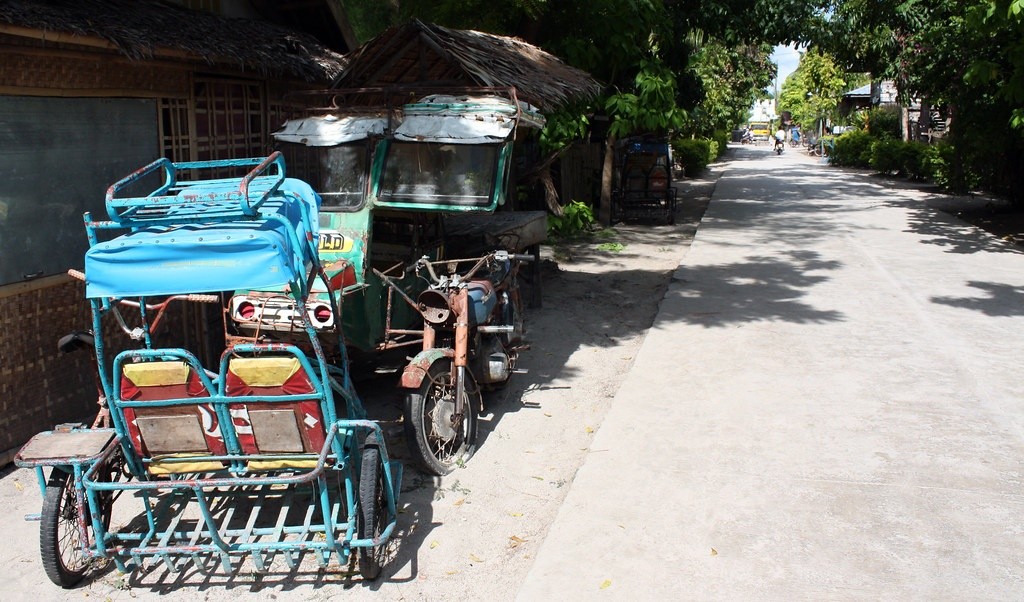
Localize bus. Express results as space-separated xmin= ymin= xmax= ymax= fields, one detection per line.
xmin=749 ymin=122 xmax=770 ymax=141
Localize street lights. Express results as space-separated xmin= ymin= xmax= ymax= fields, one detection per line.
xmin=807 ymin=91 xmax=826 ymax=162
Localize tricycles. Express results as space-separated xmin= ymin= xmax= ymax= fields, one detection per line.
xmin=612 ymin=136 xmax=679 ymax=225
xmin=12 ymin=150 xmax=407 ymax=588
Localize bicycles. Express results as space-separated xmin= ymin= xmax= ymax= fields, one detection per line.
xmin=807 ymin=143 xmax=826 ymax=157
xmin=57 ymin=269 xmax=221 ymax=428
xmin=788 ymin=133 xmax=810 ymax=148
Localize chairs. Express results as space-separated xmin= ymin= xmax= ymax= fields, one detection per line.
xmin=113 ymin=343 xmax=347 ymax=480
xmin=622 ymin=164 xmax=669 ymax=206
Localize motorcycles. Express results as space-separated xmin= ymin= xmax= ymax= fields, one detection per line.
xmin=772 ymin=135 xmax=785 ymax=155
xmin=262 ymin=84 xmax=547 ymax=477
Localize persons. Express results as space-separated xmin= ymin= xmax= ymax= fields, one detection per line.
xmin=774 ymin=126 xmax=786 ymax=152
xmin=740 ymin=126 xmax=800 ymax=147
